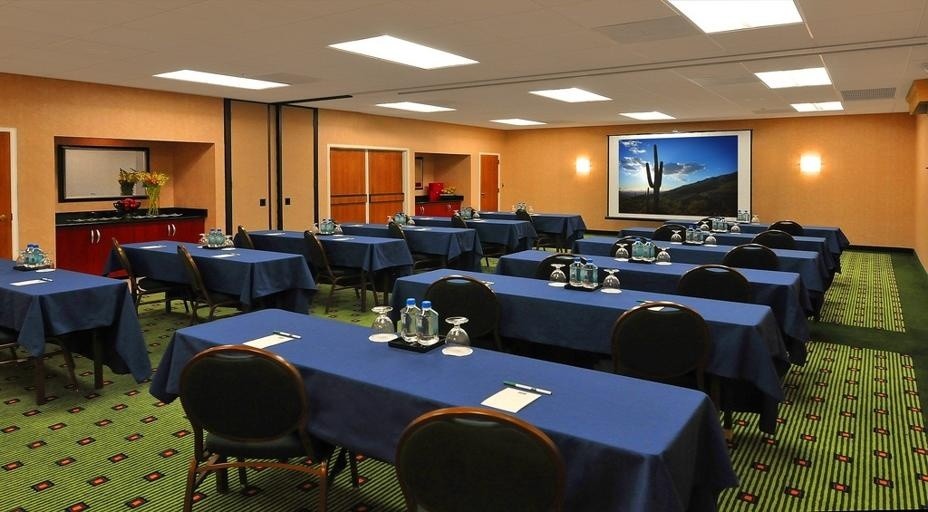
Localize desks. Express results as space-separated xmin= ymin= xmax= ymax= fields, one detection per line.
xmin=233 ymin=231 xmax=414 ymax=306
xmin=102 ymin=240 xmax=318 ymax=315
xmin=499 ymin=250 xmax=807 ymax=361
xmin=575 ymin=238 xmax=829 ymax=324
xmin=0 ymin=258 xmax=152 ymax=405
xmin=386 ymin=268 xmax=791 ymax=435
xmin=148 ymin=309 xmax=740 ymax=511
xmin=619 ymin=227 xmax=834 ymax=301
xmin=666 ymin=219 xmax=848 ymax=277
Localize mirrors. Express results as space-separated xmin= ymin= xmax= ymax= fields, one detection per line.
xmin=55 ymin=144 xmax=151 ymax=203
xmin=414 ymin=156 xmax=423 ymax=190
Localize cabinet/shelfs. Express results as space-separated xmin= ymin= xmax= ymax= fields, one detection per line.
xmin=131 ymin=219 xmax=204 ymax=243
xmin=415 ymin=201 xmax=460 ymax=218
xmin=56 ymin=223 xmax=131 ymax=279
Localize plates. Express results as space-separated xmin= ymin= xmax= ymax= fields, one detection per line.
xmin=439 ymin=193 xmax=455 ymax=197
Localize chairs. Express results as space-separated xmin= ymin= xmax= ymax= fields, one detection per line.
xmin=751 ymin=230 xmax=797 ymax=250
xmin=534 ymin=253 xmax=588 ymax=283
xmin=236 ymin=225 xmax=256 ymax=249
xmin=176 ymin=246 xmax=249 ymax=325
xmin=651 ymin=224 xmax=688 ymax=242
xmin=609 ymin=300 xmax=713 ymax=393
xmin=610 ymin=236 xmax=660 ymax=258
xmin=393 ymin=406 xmax=566 ymax=511
xmin=421 ymin=274 xmax=504 ymax=352
xmin=722 ymin=243 xmax=780 ymax=271
xmin=676 ymin=265 xmax=752 ymax=304
xmin=766 ymin=220 xmax=804 ymax=236
xmin=696 ymin=218 xmax=731 ymax=231
xmin=329 ymin=208 xmax=586 ymax=270
xmin=303 ymin=229 xmax=379 ymax=314
xmin=111 ymin=236 xmax=190 ymax=316
xmin=178 ymin=344 xmax=360 ymax=511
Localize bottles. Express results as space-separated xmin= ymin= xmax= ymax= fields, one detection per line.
xmin=736 ymin=210 xmax=759 ymax=225
xmin=579 ymin=258 xmax=598 ymax=290
xmin=510 ymin=202 xmax=534 ymax=215
xmin=452 ymin=208 xmax=481 ymax=221
xmin=692 ymin=229 xmax=703 ymax=244
xmin=685 ymin=228 xmax=695 ymax=243
xmin=198 ymin=227 xmax=234 ymax=249
xmin=415 ymin=300 xmax=439 ymax=347
xmin=386 ymin=213 xmax=415 ymax=228
xmin=642 ymin=238 xmax=655 ymax=262
xmin=399 ymin=297 xmax=422 ymax=344
xmin=568 ymin=256 xmax=583 ymax=288
xmin=630 ymin=237 xmax=643 ymax=261
xmin=15 ymin=244 xmax=56 ymax=271
xmin=699 ymin=217 xmax=741 ymax=233
xmin=309 ymin=218 xmax=343 ymax=237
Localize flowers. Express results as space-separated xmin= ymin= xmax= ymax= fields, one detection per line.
xmin=134 ymin=171 xmax=169 ymax=186
xmin=117 ymin=170 xmax=136 ymax=183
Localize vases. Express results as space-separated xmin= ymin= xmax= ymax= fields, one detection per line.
xmin=120 ymin=183 xmax=134 ymax=195
xmin=144 ymin=186 xmax=161 ymax=216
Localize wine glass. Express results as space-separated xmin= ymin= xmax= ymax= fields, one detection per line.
xmin=368 ymin=305 xmax=397 ymax=343
xmin=440 ymin=316 xmax=472 ymax=356
xmin=600 ymin=268 xmax=621 ymax=293
xmin=670 ymin=228 xmax=681 ymax=244
xmin=655 ymin=245 xmax=671 ymax=264
xmin=547 ymin=263 xmax=566 ymax=287
xmin=614 ymin=243 xmax=630 ymax=261
xmin=702 ymin=233 xmax=716 ymax=247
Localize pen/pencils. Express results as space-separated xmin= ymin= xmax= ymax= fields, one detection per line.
xmin=272 ymin=331 xmax=302 ymax=339
xmin=40 ymin=277 xmax=54 ymax=282
xmin=465 ymin=280 xmax=495 ymax=285
xmin=503 ymin=381 xmax=552 ymax=395
xmin=636 ymin=301 xmax=650 ymax=303
